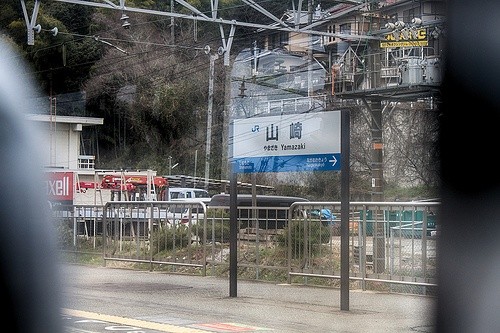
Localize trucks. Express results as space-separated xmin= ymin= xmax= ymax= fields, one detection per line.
xmin=52 ymin=187 xmax=212 ymax=231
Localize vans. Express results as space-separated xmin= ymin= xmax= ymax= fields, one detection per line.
xmin=206 ymin=193 xmax=331 ymax=244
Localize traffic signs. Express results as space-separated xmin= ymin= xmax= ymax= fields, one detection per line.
xmin=233 ymin=110 xmax=345 ymax=171
